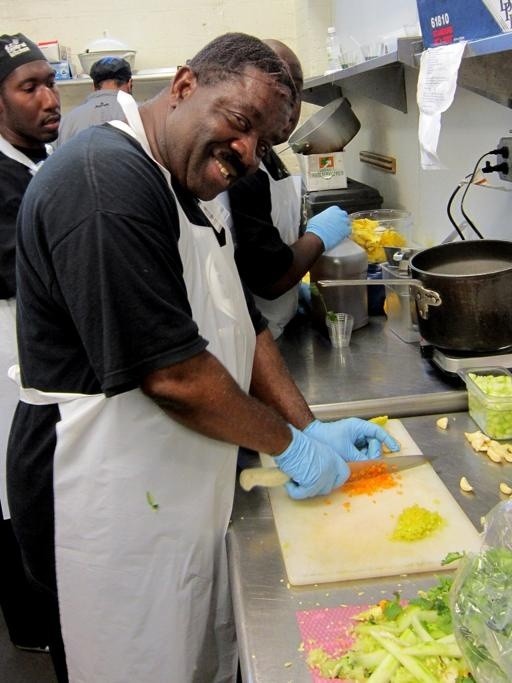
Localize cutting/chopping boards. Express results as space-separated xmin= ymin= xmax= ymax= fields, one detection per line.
xmin=257 ymin=416 xmax=490 ymax=587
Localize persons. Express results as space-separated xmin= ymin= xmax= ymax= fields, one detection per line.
xmin=4 ymin=29 xmax=403 ymax=682
xmin=51 ymin=55 xmax=135 ymax=151
xmin=0 ymin=29 xmax=64 ymax=653
xmin=221 ymin=38 xmax=354 ymax=339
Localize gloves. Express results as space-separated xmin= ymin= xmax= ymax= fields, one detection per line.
xmin=304 ymin=206 xmax=352 ymax=251
xmin=272 ymin=422 xmax=349 ymax=498
xmin=302 ymin=417 xmax=401 ymax=461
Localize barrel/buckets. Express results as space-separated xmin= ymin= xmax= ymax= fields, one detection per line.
xmin=310 ymin=236 xmax=369 ymax=332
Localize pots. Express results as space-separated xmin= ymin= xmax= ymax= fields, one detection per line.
xmin=77 ymin=32 xmax=138 ymax=76
xmin=317 ymin=238 xmax=512 ymax=353
xmin=275 ymin=95 xmax=363 ymax=155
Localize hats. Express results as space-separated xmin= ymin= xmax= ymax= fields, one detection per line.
xmin=0 ymin=33 xmax=47 ymax=81
xmin=89 ymin=57 xmax=131 ymax=82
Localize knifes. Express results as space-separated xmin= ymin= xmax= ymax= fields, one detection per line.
xmin=238 ymin=454 xmax=440 ymax=493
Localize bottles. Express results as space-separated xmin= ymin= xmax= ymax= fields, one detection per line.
xmin=326 ymin=26 xmax=341 ymax=72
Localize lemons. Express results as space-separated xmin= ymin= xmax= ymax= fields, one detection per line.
xmin=384 ymin=292 xmax=400 ymax=318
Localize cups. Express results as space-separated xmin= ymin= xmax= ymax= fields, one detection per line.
xmin=325 ymin=312 xmax=354 ymax=349
